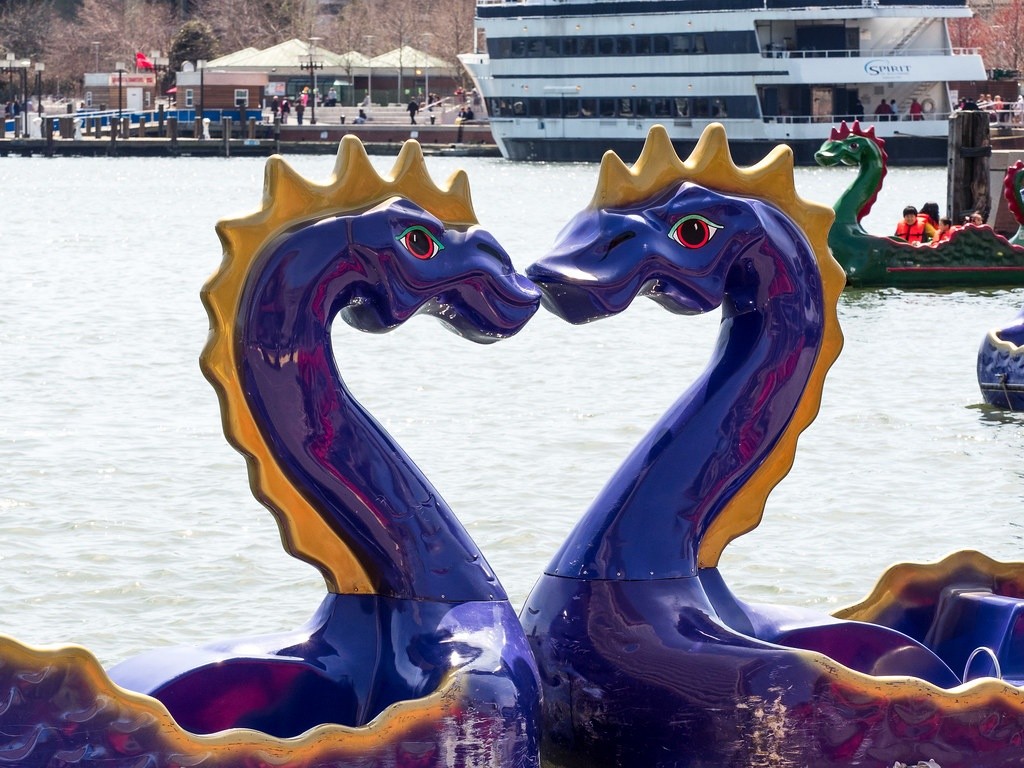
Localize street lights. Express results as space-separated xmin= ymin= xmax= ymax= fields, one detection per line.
xmin=197 ymin=60 xmax=208 ymax=140
xmin=35 ymin=62 xmax=45 ymax=136
xmin=363 ymin=35 xmax=376 ymax=121
xmin=91 ymin=41 xmax=102 ymax=73
xmin=115 ymin=62 xmax=125 ymax=138
xmin=308 ymin=36 xmax=325 ymax=120
xmin=419 ymin=31 xmax=432 ymax=124
xmin=6 ymin=53 xmax=15 ymax=118
xmin=151 ymin=51 xmax=160 ymax=72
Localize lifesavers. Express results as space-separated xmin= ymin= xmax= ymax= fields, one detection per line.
xmin=922 ymin=98 xmax=936 ymax=113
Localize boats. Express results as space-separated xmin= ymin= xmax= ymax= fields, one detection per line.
xmin=516 ymin=122 xmax=1024 ymax=768
xmin=977 ymin=303 xmax=1024 ymax=412
xmin=815 ymin=119 xmax=1024 ymax=288
xmin=0 ymin=136 xmax=543 ymax=768
xmin=456 ymin=0 xmax=989 ymax=165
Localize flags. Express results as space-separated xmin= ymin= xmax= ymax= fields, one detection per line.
xmin=135 ymin=52 xmax=153 ymax=68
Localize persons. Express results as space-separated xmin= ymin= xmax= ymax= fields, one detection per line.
xmin=297 ymin=87 xmax=337 ymax=107
xmin=295 ymin=101 xmax=304 ymax=125
xmin=455 ymin=108 xmax=466 ymax=125
xmin=462 ymin=107 xmax=473 ymax=121
xmin=960 ymin=214 xmax=982 ymax=230
xmin=855 ymin=100 xmax=864 ymax=122
xmin=910 ymin=98 xmax=922 ymax=121
xmin=416 ymin=92 xmax=441 ymax=112
xmin=271 ymin=95 xmax=290 ymax=124
xmin=894 ymin=206 xmax=938 ymax=247
xmin=352 ymin=110 xmax=366 ymax=124
xmin=454 ymin=86 xmax=465 ymax=103
xmin=931 ymin=220 xmax=956 ymax=246
xmin=360 ymin=93 xmax=369 ymax=107
xmin=406 ymin=96 xmax=419 ymax=125
xmin=5 ymin=96 xmax=19 ymax=119
xmin=953 ymin=94 xmax=1024 ymax=127
xmin=917 ymin=203 xmax=940 ymax=231
xmin=890 ymin=99 xmax=898 ymax=121
xmin=875 ymin=99 xmax=892 ymax=121
